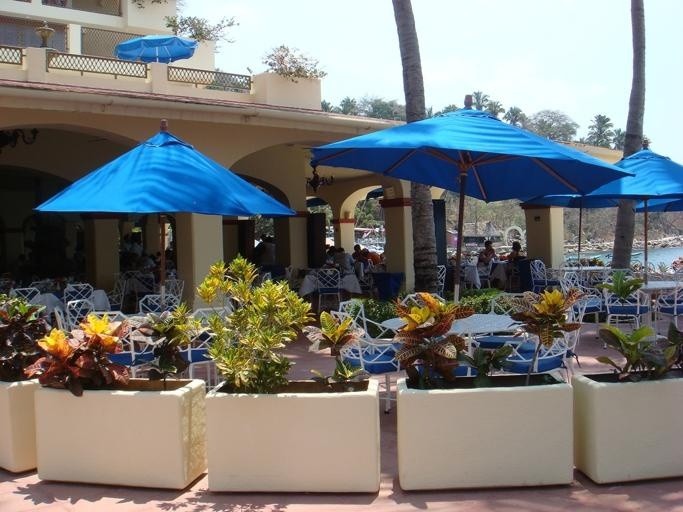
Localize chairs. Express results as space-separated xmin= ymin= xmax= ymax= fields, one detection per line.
xmin=449 ymin=255 xmax=535 ymax=290
xmin=285 ymin=259 xmax=388 ymax=313
xmin=190 ymin=308 xmax=238 ymax=388
xmin=426 ymin=266 xmax=447 ymax=299
xmin=9 ymin=262 xmax=183 ymax=378
xmin=530 ymin=254 xmax=683 ymax=348
xmin=339 ymin=298 xmax=587 ymax=412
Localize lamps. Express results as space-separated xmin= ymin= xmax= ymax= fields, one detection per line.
xmin=302 ymin=165 xmax=337 ymax=193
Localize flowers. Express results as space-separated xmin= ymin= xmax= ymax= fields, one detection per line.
xmin=266 ymin=45 xmax=328 ymax=81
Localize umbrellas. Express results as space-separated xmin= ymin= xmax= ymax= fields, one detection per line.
xmin=31 ymin=121 xmax=297 ymax=311
xmin=575 ymin=140 xmax=683 ymax=285
xmin=113 ymin=33 xmax=198 ymax=63
xmin=305 ymin=96 xmax=635 ymax=303
xmin=519 ymin=195 xmax=620 ymax=269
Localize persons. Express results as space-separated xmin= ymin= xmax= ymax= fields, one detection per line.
xmin=325 ymin=244 xmax=381 ymax=274
xmin=478 ymin=240 xmax=498 ymax=264
xmin=9 ymin=253 xmax=27 ymax=277
xmin=122 ymin=232 xmax=176 ymax=270
xmin=502 ymin=241 xmax=528 ymax=261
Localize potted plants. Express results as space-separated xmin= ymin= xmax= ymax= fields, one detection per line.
xmin=1 ymin=295 xmax=55 ymax=471
xmin=571 ymin=272 xmax=683 ymax=484
xmin=394 ymin=286 xmax=586 ymax=489
xmin=196 ymin=256 xmax=381 ymax=493
xmin=23 ymin=305 xmax=207 ymax=490
